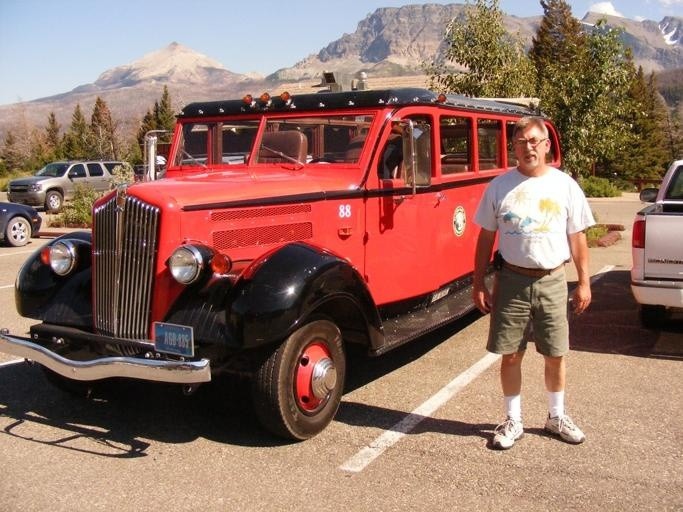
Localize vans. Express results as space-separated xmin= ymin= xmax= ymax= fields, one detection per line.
xmin=6 ymin=160 xmax=138 ymax=214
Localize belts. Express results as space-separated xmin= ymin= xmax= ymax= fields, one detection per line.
xmin=504 ymin=259 xmax=565 ymax=277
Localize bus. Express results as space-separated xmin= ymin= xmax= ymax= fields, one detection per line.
xmin=0 ymin=71 xmax=568 ymax=443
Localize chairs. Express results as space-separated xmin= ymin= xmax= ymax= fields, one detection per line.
xmin=242 ymin=130 xmax=404 ymax=181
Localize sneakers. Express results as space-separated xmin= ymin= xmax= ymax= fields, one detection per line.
xmin=495 ymin=419 xmax=526 ymax=450
xmin=543 ymin=412 xmax=587 ymax=443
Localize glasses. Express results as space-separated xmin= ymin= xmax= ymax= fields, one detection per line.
xmin=515 ymin=136 xmax=548 ymax=148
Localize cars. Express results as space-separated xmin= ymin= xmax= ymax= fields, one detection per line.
xmin=0 ymin=201 xmax=41 ymax=248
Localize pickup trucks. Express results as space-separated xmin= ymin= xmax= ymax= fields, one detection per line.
xmin=631 ymin=161 xmax=682 ymax=329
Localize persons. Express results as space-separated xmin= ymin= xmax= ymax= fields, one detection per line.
xmin=471 ymin=113 xmax=596 ymax=449
